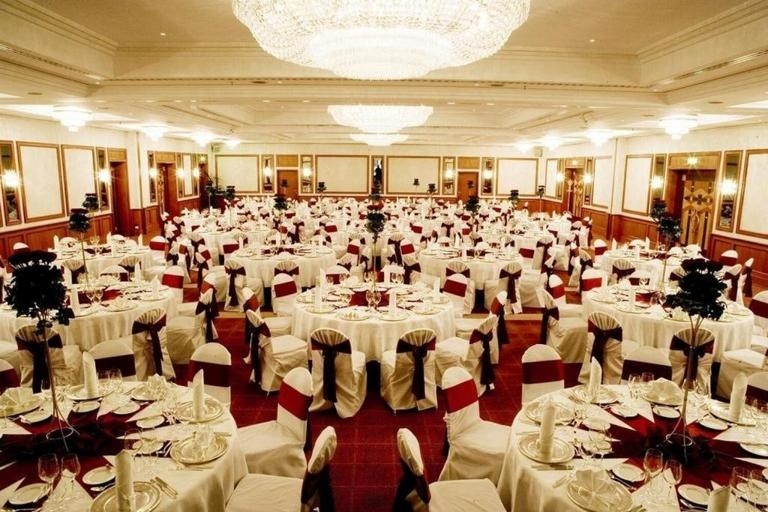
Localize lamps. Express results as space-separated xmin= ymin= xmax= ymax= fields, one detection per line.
xmin=327 ymin=105 xmax=433 ymax=132
xmin=230 ymin=0 xmax=532 ymax=81
xmin=349 ymin=134 xmax=409 ymax=146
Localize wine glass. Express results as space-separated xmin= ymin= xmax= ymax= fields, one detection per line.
xmin=639 ymin=273 xmax=678 ymax=307
xmin=559 ymin=371 xmax=768 ymax=512
xmin=37 ymin=368 xmax=178 ymax=508
xmin=85 ymin=288 xmax=104 ymax=304
xmin=340 ymin=271 xmax=403 ymax=311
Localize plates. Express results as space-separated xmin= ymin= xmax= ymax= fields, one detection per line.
xmin=517 ymin=387 xmax=768 ymax=512
xmin=590 ymin=285 xmax=751 ymax=323
xmin=68 ymin=281 xmax=170 ymax=318
xmin=297 ymin=281 xmax=451 ymax=321
xmin=1 ymin=377 xmax=230 ymax=512
